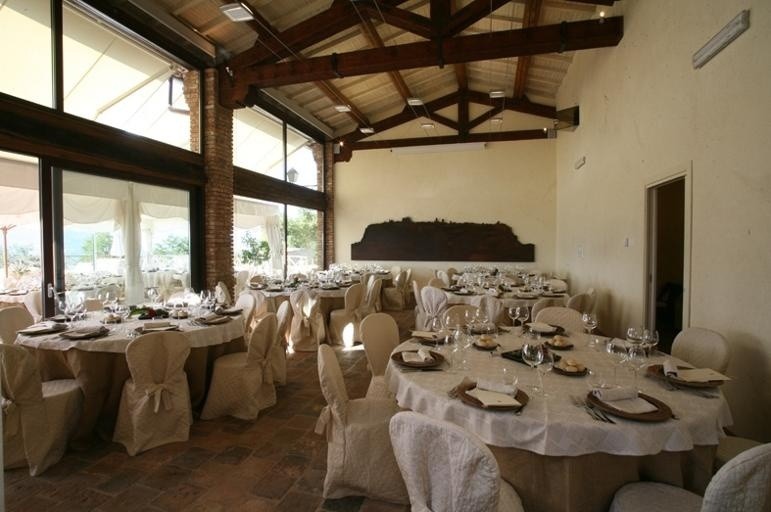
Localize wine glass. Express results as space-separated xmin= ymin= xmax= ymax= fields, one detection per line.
xmin=607 ymin=343 xmax=628 ymax=383
xmin=627 ymin=327 xmax=643 ymax=352
xmin=328 ymin=261 xmax=392 ymax=281
xmin=391 ymin=300 xmax=588 ymax=400
xmin=583 ymin=313 xmax=598 ymax=341
xmin=17 ymin=285 xmax=246 ymax=342
xmin=644 ymin=329 xmax=660 ymax=353
xmin=627 ymin=349 xmax=646 ymax=382
xmin=588 ymin=367 xmax=607 ymax=391
xmin=458 ymin=262 xmax=557 ymax=299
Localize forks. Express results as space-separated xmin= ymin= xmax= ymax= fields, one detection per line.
xmin=567 ymin=393 xmax=617 ymax=426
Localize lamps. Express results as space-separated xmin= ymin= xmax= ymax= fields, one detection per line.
xmin=692 ymin=9 xmax=749 ymax=70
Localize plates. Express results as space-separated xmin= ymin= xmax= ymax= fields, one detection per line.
xmin=249 ymin=279 xmax=324 ymax=293
xmin=459 ymin=381 xmax=530 ymax=413
xmin=587 ymin=387 xmax=673 ymax=421
xmin=648 ymin=364 xmax=725 ymax=388
